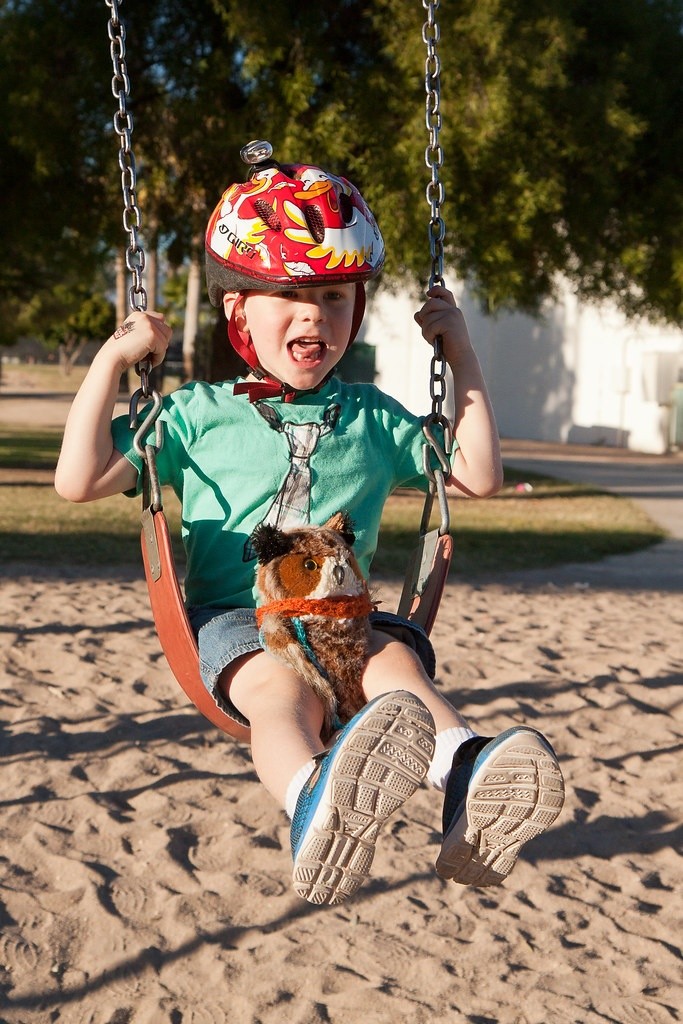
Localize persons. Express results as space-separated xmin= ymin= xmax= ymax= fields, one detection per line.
xmin=53 ymin=162 xmax=563 ymax=907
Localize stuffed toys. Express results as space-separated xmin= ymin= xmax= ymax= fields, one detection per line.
xmin=253 ymin=512 xmax=373 ymax=729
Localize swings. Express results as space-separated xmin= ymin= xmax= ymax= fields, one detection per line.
xmin=102 ymin=0 xmax=459 ymax=747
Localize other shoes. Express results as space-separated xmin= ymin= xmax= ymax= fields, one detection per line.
xmin=288 ymin=688 xmax=436 ymax=906
xmin=438 ymin=726 xmax=562 ymax=886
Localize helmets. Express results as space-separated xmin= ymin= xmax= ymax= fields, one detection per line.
xmin=204 ymin=159 xmax=385 ymax=306
